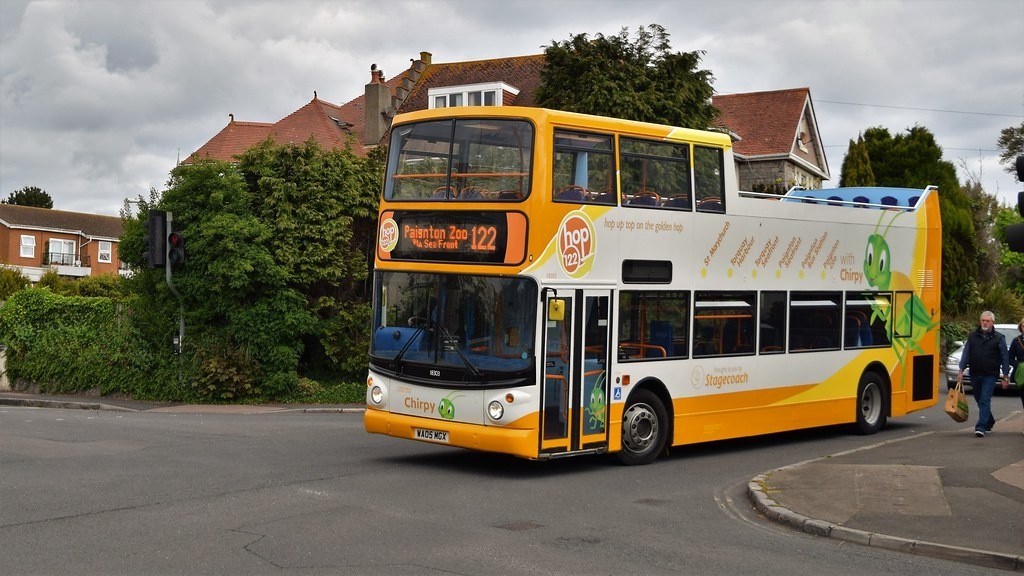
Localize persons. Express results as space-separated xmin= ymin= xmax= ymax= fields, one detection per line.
xmin=1002 ymin=318 xmax=1024 ymax=409
xmin=957 ymin=311 xmax=1009 ymax=437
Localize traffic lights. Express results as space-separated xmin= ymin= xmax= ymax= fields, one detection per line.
xmin=143 ymin=209 xmax=164 ymax=267
xmin=168 ymin=232 xmax=185 ymax=265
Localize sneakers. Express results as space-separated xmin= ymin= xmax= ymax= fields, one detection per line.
xmin=985 ymin=420 xmax=995 ymax=433
xmin=975 ymin=430 xmax=985 ymax=437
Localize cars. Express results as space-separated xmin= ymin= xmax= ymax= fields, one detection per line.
xmin=944 ymin=324 xmax=1018 ymax=391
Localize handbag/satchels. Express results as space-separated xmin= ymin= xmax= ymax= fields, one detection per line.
xmin=944 ymin=381 xmax=969 ymax=423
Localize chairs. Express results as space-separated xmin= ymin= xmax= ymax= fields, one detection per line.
xmin=791 ymin=310 xmax=873 ymax=349
xmin=801 ymin=195 xmax=920 ymax=212
xmin=622 ymin=320 xmax=783 ymax=356
xmin=431 ymin=185 xmax=525 ymax=200
xmin=556 ymin=185 xmax=723 ymax=211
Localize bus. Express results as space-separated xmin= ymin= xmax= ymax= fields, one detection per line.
xmin=364 ymin=105 xmax=941 ymax=462
